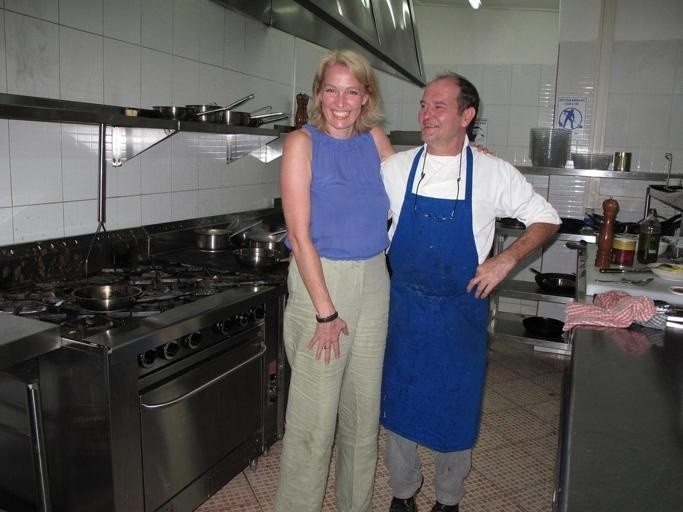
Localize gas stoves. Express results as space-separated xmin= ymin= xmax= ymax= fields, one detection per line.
xmin=0 ymin=262 xmax=264 ymax=339
xmin=211 ymin=0 xmax=430 ymax=89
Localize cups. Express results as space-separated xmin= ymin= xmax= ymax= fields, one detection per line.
xmin=614 ymin=152 xmax=632 ymax=171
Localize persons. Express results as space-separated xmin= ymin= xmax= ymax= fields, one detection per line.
xmin=270 ymin=46 xmax=395 ymax=512
xmin=562 ymin=109 xmax=576 ymax=128
xmin=380 ymin=72 xmax=562 ymax=512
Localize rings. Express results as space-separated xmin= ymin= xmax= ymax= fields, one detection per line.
xmin=323 ymin=343 xmax=331 ymax=351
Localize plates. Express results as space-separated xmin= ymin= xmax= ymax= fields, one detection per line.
xmin=668 ymin=285 xmax=683 ymax=295
xmin=520 ymin=315 xmax=564 ymax=336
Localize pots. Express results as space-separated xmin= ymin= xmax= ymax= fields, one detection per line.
xmin=148 ymin=92 xmax=286 ymax=136
xmin=529 ymin=267 xmax=575 ymax=295
xmin=193 ymin=217 xmax=289 ymax=265
xmin=558 ymin=211 xmax=683 ymax=236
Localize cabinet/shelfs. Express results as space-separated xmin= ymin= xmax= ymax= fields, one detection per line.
xmin=489 ymin=166 xmax=683 ymax=355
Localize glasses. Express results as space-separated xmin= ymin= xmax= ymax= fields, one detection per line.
xmin=414 ymin=174 xmax=459 ymax=222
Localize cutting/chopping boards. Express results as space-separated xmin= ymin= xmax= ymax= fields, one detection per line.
xmin=585 ymin=243 xmax=682 ymax=306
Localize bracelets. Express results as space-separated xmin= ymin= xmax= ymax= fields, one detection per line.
xmin=315 ymin=312 xmax=339 ymax=323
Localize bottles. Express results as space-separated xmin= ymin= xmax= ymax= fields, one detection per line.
xmin=636 ymin=206 xmax=660 ymax=264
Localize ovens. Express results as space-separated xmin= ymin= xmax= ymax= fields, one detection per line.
xmin=107 ymin=325 xmax=280 ymax=512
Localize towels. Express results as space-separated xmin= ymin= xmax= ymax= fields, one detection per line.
xmin=560 ymin=289 xmax=656 ymax=331
xmin=636 ymin=313 xmax=666 ymax=330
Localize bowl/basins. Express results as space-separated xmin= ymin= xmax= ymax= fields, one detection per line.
xmin=527 ymin=126 xmax=571 ymax=168
xmin=569 ymin=152 xmax=612 ymax=170
xmin=646 ymin=262 xmax=683 ymax=281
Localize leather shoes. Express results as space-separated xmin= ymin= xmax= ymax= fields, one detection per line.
xmin=389 ymin=476 xmax=459 ymax=512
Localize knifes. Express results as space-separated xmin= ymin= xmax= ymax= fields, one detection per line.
xmin=598 ymin=268 xmax=638 ymax=274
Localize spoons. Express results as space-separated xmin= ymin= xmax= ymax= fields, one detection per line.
xmin=637 ymin=264 xmax=673 ymax=270
xmin=595 ymin=278 xmax=654 ymax=286
